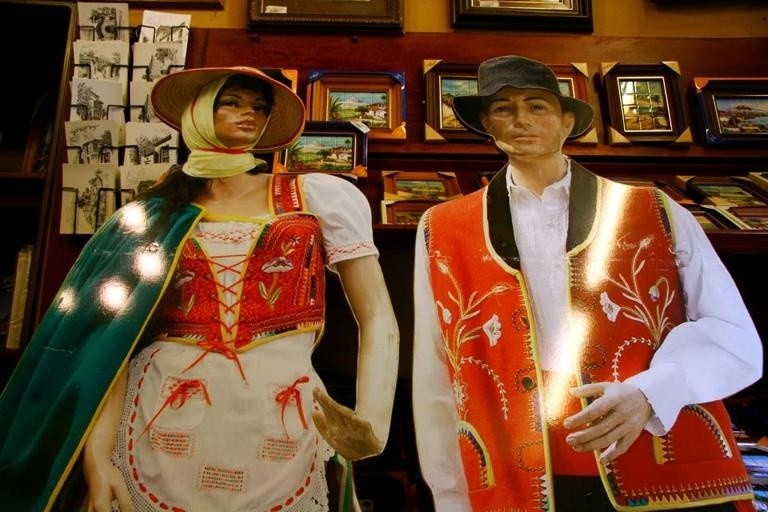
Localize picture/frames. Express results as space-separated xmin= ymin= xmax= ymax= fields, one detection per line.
xmin=382 ymin=201 xmax=447 ymax=228
xmin=304 ymin=71 xmax=406 ymax=140
xmin=272 ymin=120 xmax=369 ymax=178
xmin=614 ymin=172 xmax=768 ymax=229
xmin=602 ymin=61 xmax=694 ymax=146
xmin=695 ymin=77 xmax=765 ymax=147
xmin=424 ymin=63 xmax=598 ymax=145
xmin=382 ymin=170 xmax=462 ymax=200
xmin=446 ymin=0 xmax=593 ymax=36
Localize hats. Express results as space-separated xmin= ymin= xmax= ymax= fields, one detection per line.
xmin=447 ymin=56 xmax=597 ymax=143
xmin=143 ymin=63 xmax=310 ymax=152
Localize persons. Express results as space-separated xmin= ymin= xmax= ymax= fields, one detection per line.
xmin=0 ymin=63 xmax=402 ymax=512
xmin=410 ymin=53 xmax=768 ymax=512
